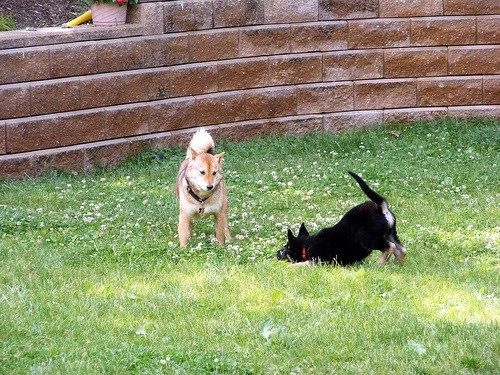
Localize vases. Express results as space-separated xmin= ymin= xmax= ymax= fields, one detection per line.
xmin=91 ymin=2 xmax=127 ymax=26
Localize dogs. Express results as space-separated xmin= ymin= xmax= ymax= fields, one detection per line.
xmin=276 ymin=170 xmax=408 ymax=266
xmin=177 ymin=128 xmax=232 ymax=249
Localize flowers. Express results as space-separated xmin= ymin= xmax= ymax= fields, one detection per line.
xmin=86 ymin=0 xmax=138 ymax=7
